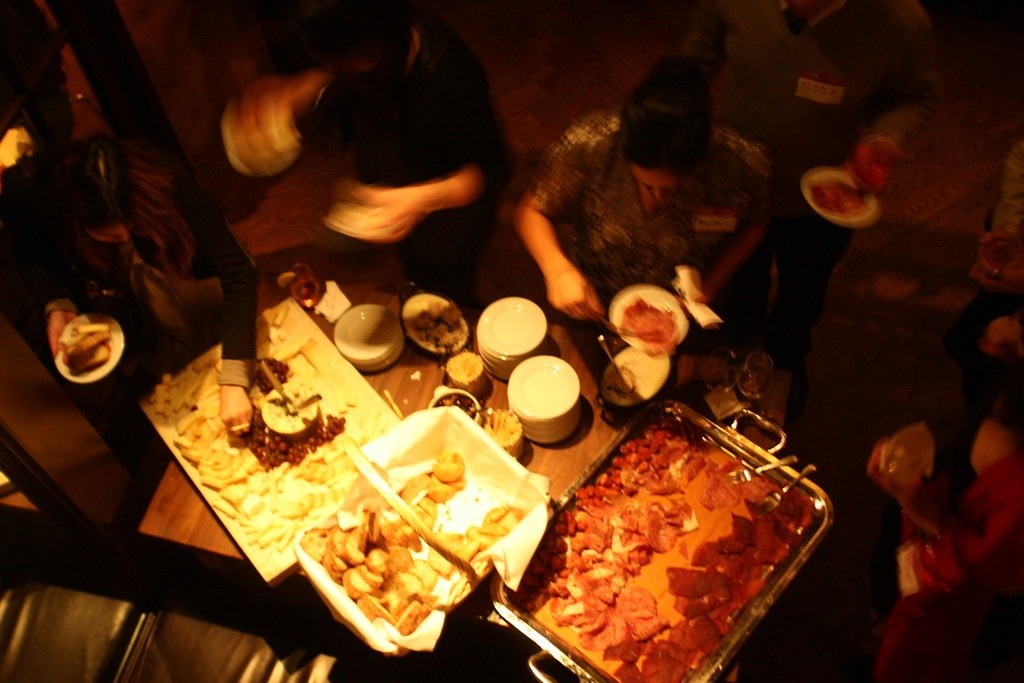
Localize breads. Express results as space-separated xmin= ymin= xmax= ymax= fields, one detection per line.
xmin=66 ymin=329 xmax=113 ymax=372
xmin=303 ymin=449 xmax=525 ymax=635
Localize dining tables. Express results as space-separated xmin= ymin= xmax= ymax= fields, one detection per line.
xmin=138 ymin=285 xmax=791 ymax=683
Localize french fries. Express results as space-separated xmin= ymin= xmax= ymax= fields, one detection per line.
xmin=145 ymin=364 xmax=364 ymax=551
xmin=72 ymin=323 xmax=111 ymax=335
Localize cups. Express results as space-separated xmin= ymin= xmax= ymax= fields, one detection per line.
xmin=704 ymin=348 xmax=776 ymax=401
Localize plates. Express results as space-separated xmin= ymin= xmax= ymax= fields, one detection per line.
xmin=605 ymin=347 xmax=670 ymax=405
xmin=262 ymin=382 xmax=319 ymax=438
xmin=53 ymin=314 xmax=124 ymax=382
xmin=507 ymin=356 xmax=581 ymax=444
xmin=476 ymin=297 xmax=551 ymax=381
xmin=334 ymin=304 xmax=405 ymax=372
xmin=801 ymin=167 xmax=881 ymax=229
xmin=404 ymin=294 xmax=470 ymax=352
xmin=610 ymin=285 xmax=687 ymax=349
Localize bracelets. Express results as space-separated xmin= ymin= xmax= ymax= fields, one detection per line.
xmin=67 ymin=92 xmax=94 ymax=108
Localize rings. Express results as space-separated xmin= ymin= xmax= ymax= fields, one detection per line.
xmin=231 ymin=422 xmax=251 ymax=433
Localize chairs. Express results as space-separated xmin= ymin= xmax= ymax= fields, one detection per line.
xmin=112 ymin=606 xmax=338 ymax=682
xmin=0 ymin=580 xmax=148 ymax=682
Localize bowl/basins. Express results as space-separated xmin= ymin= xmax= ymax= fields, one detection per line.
xmin=428 ymin=386 xmax=483 ymax=430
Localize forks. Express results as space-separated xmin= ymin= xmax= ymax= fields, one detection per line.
xmin=758 ymin=464 xmax=817 ymax=513
xmin=728 ymin=455 xmax=798 ymax=484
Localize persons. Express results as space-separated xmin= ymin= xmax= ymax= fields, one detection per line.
xmin=217 ymin=0 xmax=517 ymax=313
xmin=638 ymin=0 xmax=1024 ymax=683
xmin=513 ymin=53 xmax=773 ymax=353
xmin=1 ymin=129 xmax=259 ymax=438
